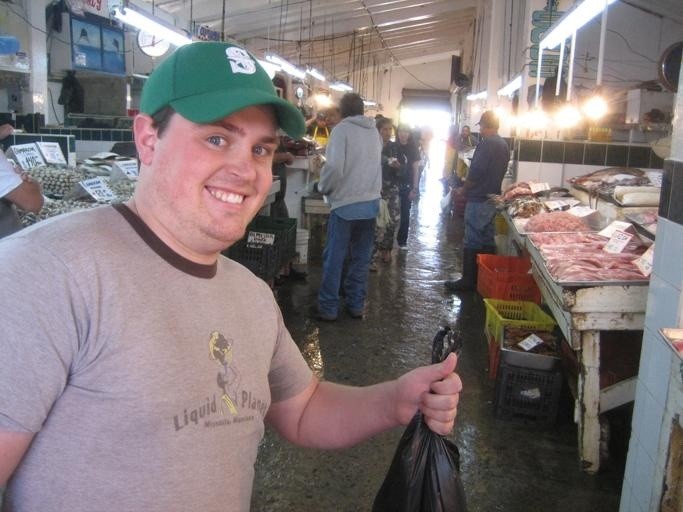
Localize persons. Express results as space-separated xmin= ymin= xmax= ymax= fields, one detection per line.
xmin=443 ymin=112 xmax=512 ymax=291
xmin=312 ymin=89 xmax=383 ymax=318
xmin=366 ymin=113 xmax=477 ymax=273
xmin=0 ymin=121 xmax=46 ymax=239
xmin=269 ymin=138 xmax=309 ymax=284
xmin=304 ymin=110 xmax=332 ymax=146
xmin=0 ymin=42 xmax=463 ymax=511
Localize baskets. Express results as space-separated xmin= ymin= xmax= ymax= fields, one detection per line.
xmin=476 ymin=253 xmax=568 ymax=428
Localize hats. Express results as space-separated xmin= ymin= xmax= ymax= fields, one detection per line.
xmin=140 ymin=42 xmax=306 ymax=139
xmin=474 ymin=111 xmax=500 ymax=128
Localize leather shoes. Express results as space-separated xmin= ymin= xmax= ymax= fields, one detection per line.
xmin=445 ymin=281 xmax=477 ymax=290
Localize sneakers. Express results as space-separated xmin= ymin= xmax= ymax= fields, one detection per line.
xmin=368 ymin=246 xmax=409 ymax=272
xmin=309 ymin=304 xmax=364 ymax=322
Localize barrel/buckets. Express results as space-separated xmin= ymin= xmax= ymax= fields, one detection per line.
xmin=296 ymin=229 xmax=309 ymax=265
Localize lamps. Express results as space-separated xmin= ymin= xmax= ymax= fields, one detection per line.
xmin=462 ymin=1 xmax=610 ymax=135
xmin=111 ymin=1 xmax=386 ymax=136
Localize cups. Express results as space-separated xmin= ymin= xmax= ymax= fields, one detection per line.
xmin=127 ymin=108 xmax=139 ymax=117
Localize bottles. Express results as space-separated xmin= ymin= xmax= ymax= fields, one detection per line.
xmin=319 ymin=153 xmax=326 ymax=168
